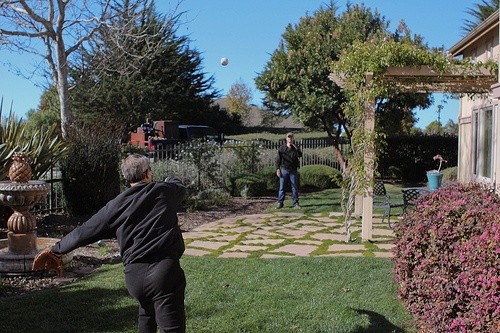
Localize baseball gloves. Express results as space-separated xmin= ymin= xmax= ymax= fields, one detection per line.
xmin=32 ymin=247 xmax=64 ymax=278
xmin=277 ymin=169 xmax=282 ymax=178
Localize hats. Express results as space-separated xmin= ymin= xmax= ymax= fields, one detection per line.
xmin=287 ymin=132 xmax=294 ymax=138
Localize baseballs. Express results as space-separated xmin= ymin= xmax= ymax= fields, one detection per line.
xmin=221 ymin=57 xmax=228 ymax=66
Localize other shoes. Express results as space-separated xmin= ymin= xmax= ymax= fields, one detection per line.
xmin=294 ymin=203 xmax=302 ymax=208
xmin=276 ymin=202 xmax=282 ymax=208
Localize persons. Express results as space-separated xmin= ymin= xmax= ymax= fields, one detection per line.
xmin=32 ymin=153 xmax=187 ymax=333
xmin=275 ymin=133 xmax=303 ymax=209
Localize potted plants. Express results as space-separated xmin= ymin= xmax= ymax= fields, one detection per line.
xmin=426 ymin=154 xmax=448 ymax=190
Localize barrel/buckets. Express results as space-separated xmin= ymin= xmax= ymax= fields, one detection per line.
xmin=426 ymin=173 xmax=443 ymax=190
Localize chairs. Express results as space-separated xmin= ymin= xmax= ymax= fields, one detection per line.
xmin=374 ymin=179 xmax=390 ymax=223
xmin=401 ymin=187 xmax=432 ymax=214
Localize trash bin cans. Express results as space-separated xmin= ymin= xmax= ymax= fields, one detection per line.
xmin=427 ymin=170 xmax=443 ymax=193
